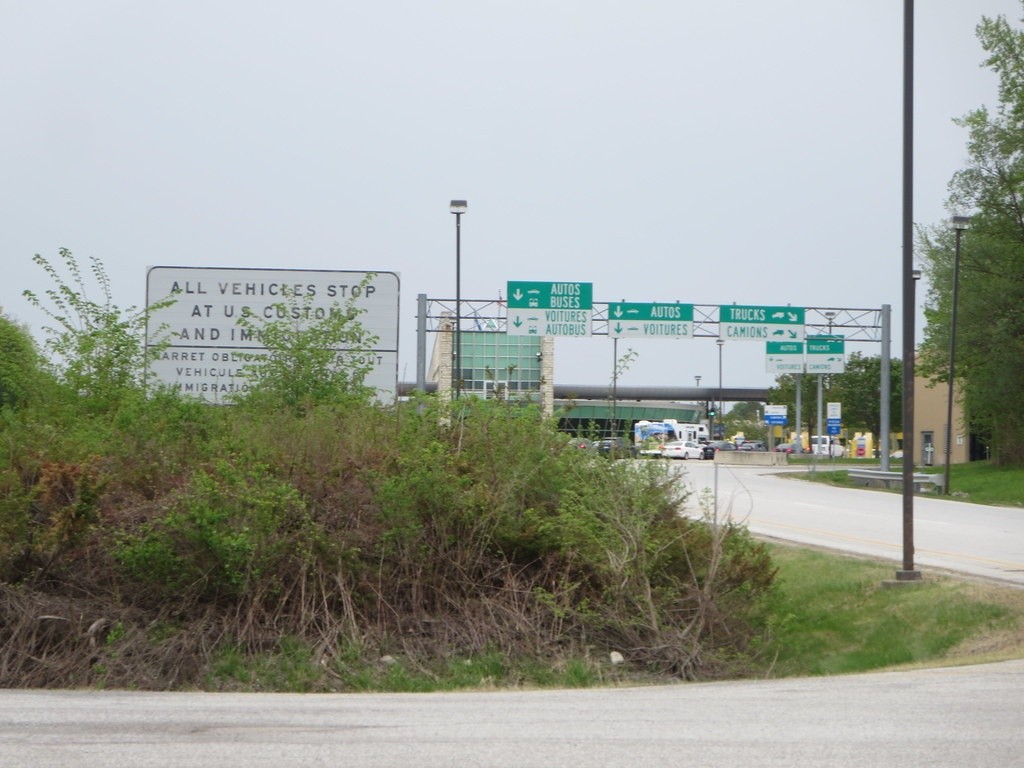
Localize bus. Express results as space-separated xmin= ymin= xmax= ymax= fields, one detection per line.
xmin=634 ymin=418 xmax=709 ymax=457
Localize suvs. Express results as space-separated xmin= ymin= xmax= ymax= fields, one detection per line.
xmin=812 ymin=435 xmax=844 ymax=458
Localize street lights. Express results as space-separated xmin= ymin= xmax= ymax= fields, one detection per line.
xmin=694 ymin=376 xmax=701 ymax=406
xmin=825 ymin=313 xmax=835 ymax=335
xmin=717 ymin=338 xmax=724 ymax=437
xmin=449 ymin=199 xmax=467 ymax=400
xmin=944 ymin=215 xmax=969 ymax=493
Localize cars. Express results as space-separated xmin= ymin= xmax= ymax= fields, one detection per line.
xmin=599 ymin=437 xmax=634 ymax=459
xmin=738 ymin=443 xmax=768 ymax=453
xmin=775 ymin=444 xmax=807 ymax=454
xmin=663 ymin=441 xmax=704 ymax=460
xmin=568 ymin=439 xmax=591 ymax=450
xmin=702 ymin=442 xmax=740 ymax=461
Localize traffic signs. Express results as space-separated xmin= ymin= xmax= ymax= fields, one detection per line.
xmin=505 ymin=278 xmax=592 ymax=340
xmin=718 ymin=307 xmax=805 ymax=342
xmin=607 ymin=301 xmax=695 ymax=340
xmin=806 ymin=335 xmax=847 ymax=373
xmin=766 ymin=343 xmax=804 ymax=376
